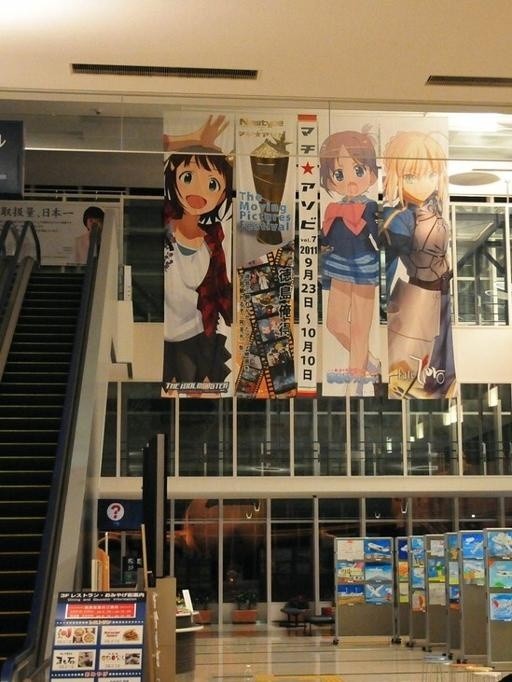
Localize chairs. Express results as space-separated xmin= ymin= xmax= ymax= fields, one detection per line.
xmin=286 ymin=597 xmax=308 ymax=621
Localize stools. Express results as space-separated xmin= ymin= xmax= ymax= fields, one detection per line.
xmin=281 ymin=607 xmax=312 ymax=627
xmin=304 ymin=616 xmax=335 ymax=636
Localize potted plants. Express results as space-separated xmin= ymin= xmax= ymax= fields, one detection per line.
xmin=193 ymin=596 xmax=216 ymax=623
xmin=231 ymin=590 xmax=256 ymax=623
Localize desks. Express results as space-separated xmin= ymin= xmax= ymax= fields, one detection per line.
xmin=176 ymin=611 xmax=204 ymax=674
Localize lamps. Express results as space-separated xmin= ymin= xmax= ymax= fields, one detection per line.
xmin=253 ymin=499 xmax=261 ymax=511
xmin=486 ymin=383 xmax=499 ymax=408
xmin=246 ymin=512 xmax=252 ymax=519
xmin=400 ymin=497 xmax=407 ymax=513
xmin=415 ymin=412 xmax=425 ymax=440
xmin=448 ymin=400 xmax=458 ymax=424
xmin=374 ymin=512 xmax=381 ymax=519
